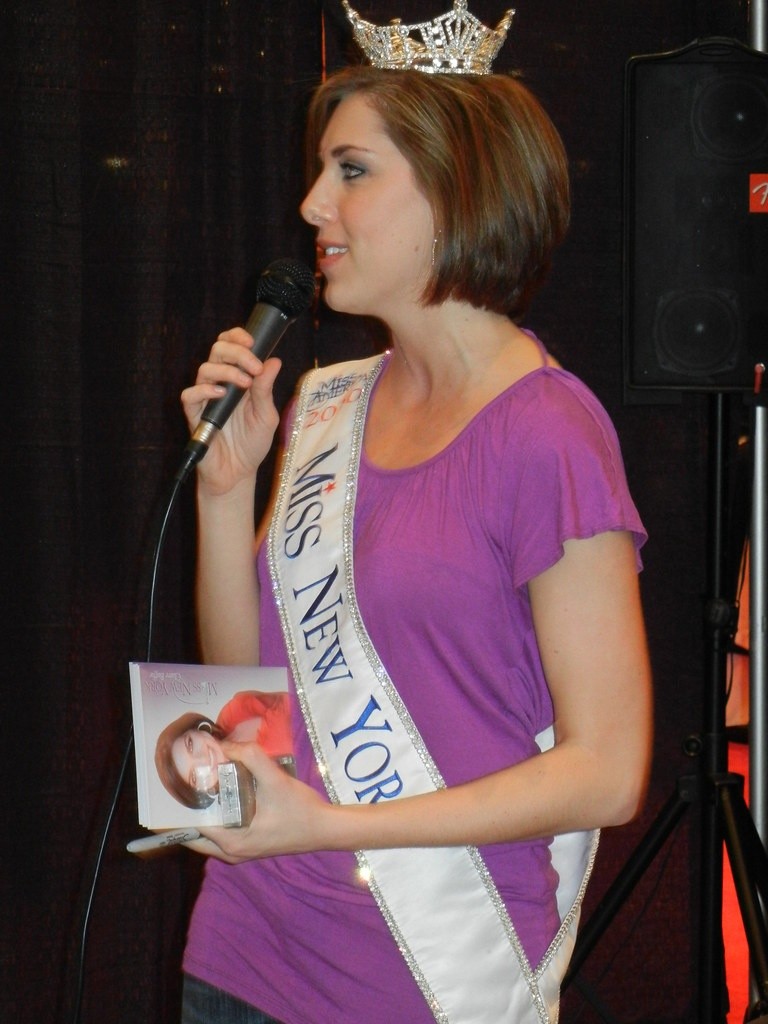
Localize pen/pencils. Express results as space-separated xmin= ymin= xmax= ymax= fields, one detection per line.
xmin=127 ymin=826 xmax=203 ymax=854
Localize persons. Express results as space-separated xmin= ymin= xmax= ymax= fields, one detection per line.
xmin=170 ymin=70 xmax=657 ymax=1021
xmin=153 ymin=688 xmax=296 ymax=811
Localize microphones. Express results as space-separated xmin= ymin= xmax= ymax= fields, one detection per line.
xmin=173 ymin=258 xmax=317 ymax=483
